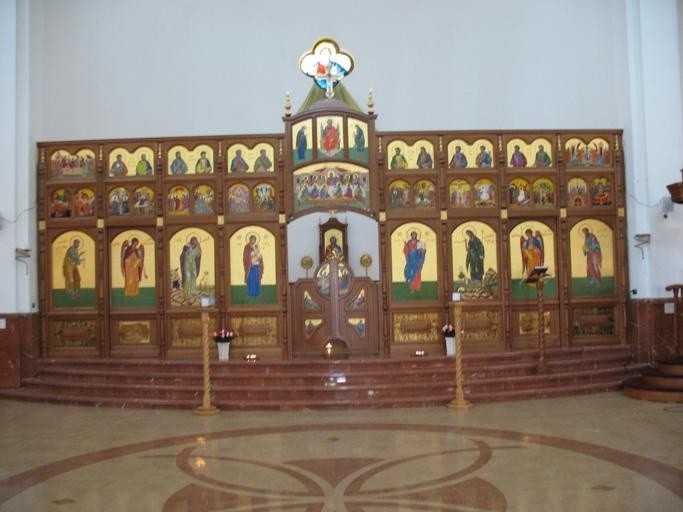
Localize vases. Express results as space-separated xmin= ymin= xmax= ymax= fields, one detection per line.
xmin=215 ymin=341 xmax=231 ymax=361
xmin=444 ymin=339 xmax=455 ymax=356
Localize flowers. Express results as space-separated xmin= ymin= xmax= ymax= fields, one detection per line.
xmin=439 ymin=324 xmax=466 ymax=338
xmin=211 ymin=327 xmax=236 ymax=342
